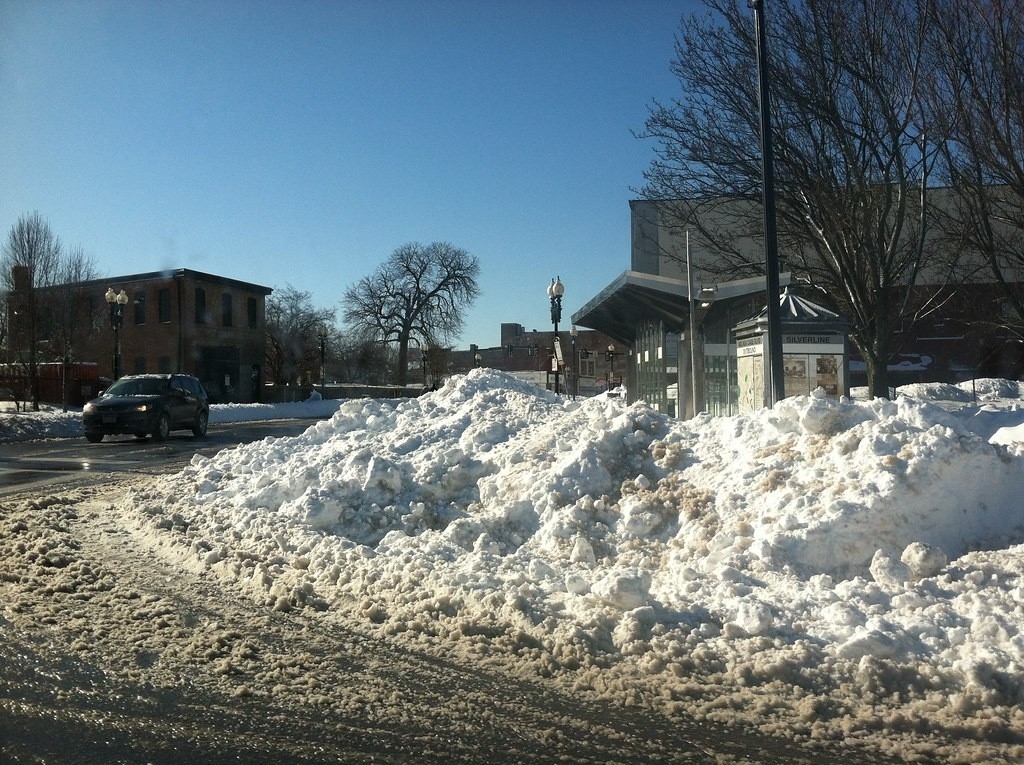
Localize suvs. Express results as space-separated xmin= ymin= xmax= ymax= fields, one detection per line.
xmin=82 ymin=372 xmax=210 ymax=442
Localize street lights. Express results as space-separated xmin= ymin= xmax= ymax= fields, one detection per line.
xmin=474 ymin=352 xmax=482 ymax=369
xmin=570 ymin=325 xmax=578 ymax=401
xmin=318 ymin=324 xmax=328 ymax=400
xmin=546 ymin=276 xmax=564 ymax=395
xmin=607 ymin=345 xmax=615 ymax=390
xmin=104 ymin=287 xmax=130 ymax=384
xmin=419 ymin=342 xmax=429 ymax=387
